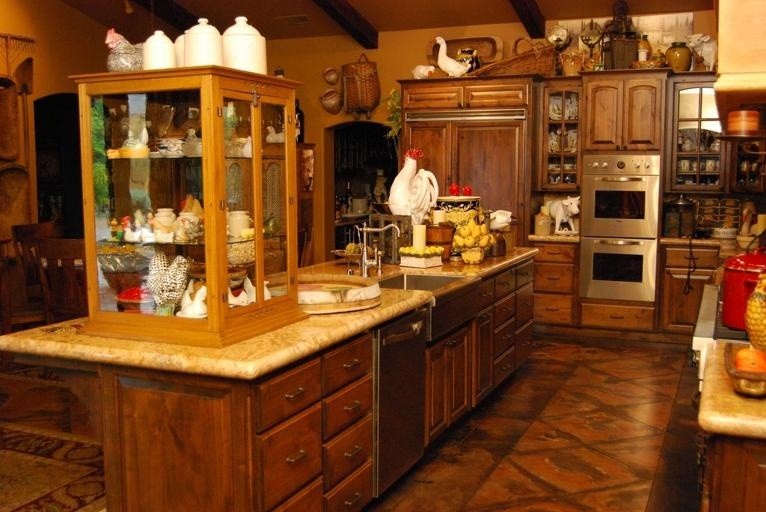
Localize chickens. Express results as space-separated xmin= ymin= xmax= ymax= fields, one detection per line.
xmin=388 ymin=147 xmax=439 ymax=227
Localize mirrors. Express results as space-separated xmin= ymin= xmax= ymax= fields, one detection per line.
xmin=579 ymin=20 xmax=603 ymax=58
xmin=546 ymin=23 xmax=570 ymax=76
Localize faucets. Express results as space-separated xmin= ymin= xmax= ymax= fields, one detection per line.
xmin=358 ymin=221 xmax=402 ymax=279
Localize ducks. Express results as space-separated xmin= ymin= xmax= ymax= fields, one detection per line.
xmin=430 ymin=35 xmax=473 ymax=78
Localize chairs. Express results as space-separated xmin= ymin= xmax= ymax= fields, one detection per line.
xmin=2 ymin=222 xmax=89 ymax=378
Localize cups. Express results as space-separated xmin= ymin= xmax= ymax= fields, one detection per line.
xmin=677 ymin=175 xmax=718 ymax=185
xmin=677 ymin=159 xmax=719 ymax=174
xmin=432 ymin=210 xmax=447 ymax=225
xmin=411 ymin=224 xmax=426 ymax=251
xmin=352 ymin=199 xmax=368 ymax=214
xmin=323 ymin=66 xmax=339 ymax=85
xmin=320 ymin=89 xmax=343 ymax=116
xmin=549 ymin=163 xmax=576 ymax=183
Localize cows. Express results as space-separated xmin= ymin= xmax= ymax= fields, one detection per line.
xmin=685 ymin=34 xmax=718 ymax=72
xmin=545 ymin=196 xmax=581 ymax=234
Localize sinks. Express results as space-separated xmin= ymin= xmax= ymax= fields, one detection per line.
xmin=376 ymin=269 xmax=467 ymax=293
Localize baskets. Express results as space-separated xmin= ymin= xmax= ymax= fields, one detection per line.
xmin=341 ymin=61 xmax=381 ymax=113
xmin=469 ymin=35 xmax=557 ymax=78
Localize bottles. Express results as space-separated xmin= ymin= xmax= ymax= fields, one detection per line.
xmin=334 ymin=179 xmax=352 ymax=223
xmin=143 ymin=15 xmax=270 ymax=73
xmin=228 ymin=210 xmax=251 ymax=235
xmin=295 ymin=97 xmax=305 ymax=141
xmin=638 ymin=34 xmax=650 ymax=61
xmin=153 ymin=208 xmax=198 ymax=241
xmin=676 ymin=128 xmax=719 ymax=152
xmin=567 ymin=93 xmax=577 ymax=119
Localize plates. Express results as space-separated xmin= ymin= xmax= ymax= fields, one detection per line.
xmin=548 ymin=96 xmax=570 ymax=121
xmin=331 ymin=249 xmax=383 ymax=259
xmin=437 ymin=196 xmax=479 ymax=202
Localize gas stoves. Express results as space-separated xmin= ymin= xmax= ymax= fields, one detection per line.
xmin=690 ymin=284 xmax=766 ymax=381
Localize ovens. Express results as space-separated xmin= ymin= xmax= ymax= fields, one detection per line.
xmin=581 ymin=176 xmax=657 ymax=299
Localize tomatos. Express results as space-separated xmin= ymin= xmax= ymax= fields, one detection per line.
xmin=462 ymin=186 xmax=472 ymax=196
xmin=449 ymin=184 xmax=460 ymax=196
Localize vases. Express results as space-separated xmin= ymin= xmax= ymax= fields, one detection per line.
xmin=664 ymin=41 xmax=692 ymax=71
xmin=636 ymin=34 xmax=652 ymax=60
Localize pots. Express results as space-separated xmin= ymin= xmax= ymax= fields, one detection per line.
xmin=721 ymin=248 xmax=765 ymax=331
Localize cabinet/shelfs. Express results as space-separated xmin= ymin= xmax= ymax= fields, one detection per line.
xmin=658 ymin=245 xmax=747 ymax=345
xmin=579 ymin=299 xmax=657 ymax=342
xmin=528 ymin=244 xmax=578 ymax=336
xmin=732 ymin=136 xmax=766 ymax=194
xmin=691 ymin=346 xmax=765 ymax=511
xmin=425 ymin=283 xmax=479 ymax=445
xmin=578 ymin=68 xmax=672 ymax=154
xmin=665 ymin=71 xmax=731 ymax=194
xmin=535 ymin=76 xmax=582 ymax=191
xmin=69 ymin=65 xmax=309 ymax=349
xmin=480 ymin=256 xmax=534 ymax=401
xmin=98 ymin=328 xmax=376 ymax=512
xmin=396 ymin=73 xmax=537 ymax=248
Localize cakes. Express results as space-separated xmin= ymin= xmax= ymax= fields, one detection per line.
xmin=399 ymin=225 xmax=445 ymax=267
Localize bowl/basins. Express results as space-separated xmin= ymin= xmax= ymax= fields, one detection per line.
xmin=736 ymin=235 xmax=759 ymax=249
xmin=105 ymin=147 xmax=163 ymax=158
xmin=371 ymin=204 xmax=391 ymax=215
xmin=710 ymin=226 xmax=737 ymax=237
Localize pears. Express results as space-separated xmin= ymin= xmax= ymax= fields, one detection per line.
xmin=452 ymin=220 xmax=496 ymax=248
